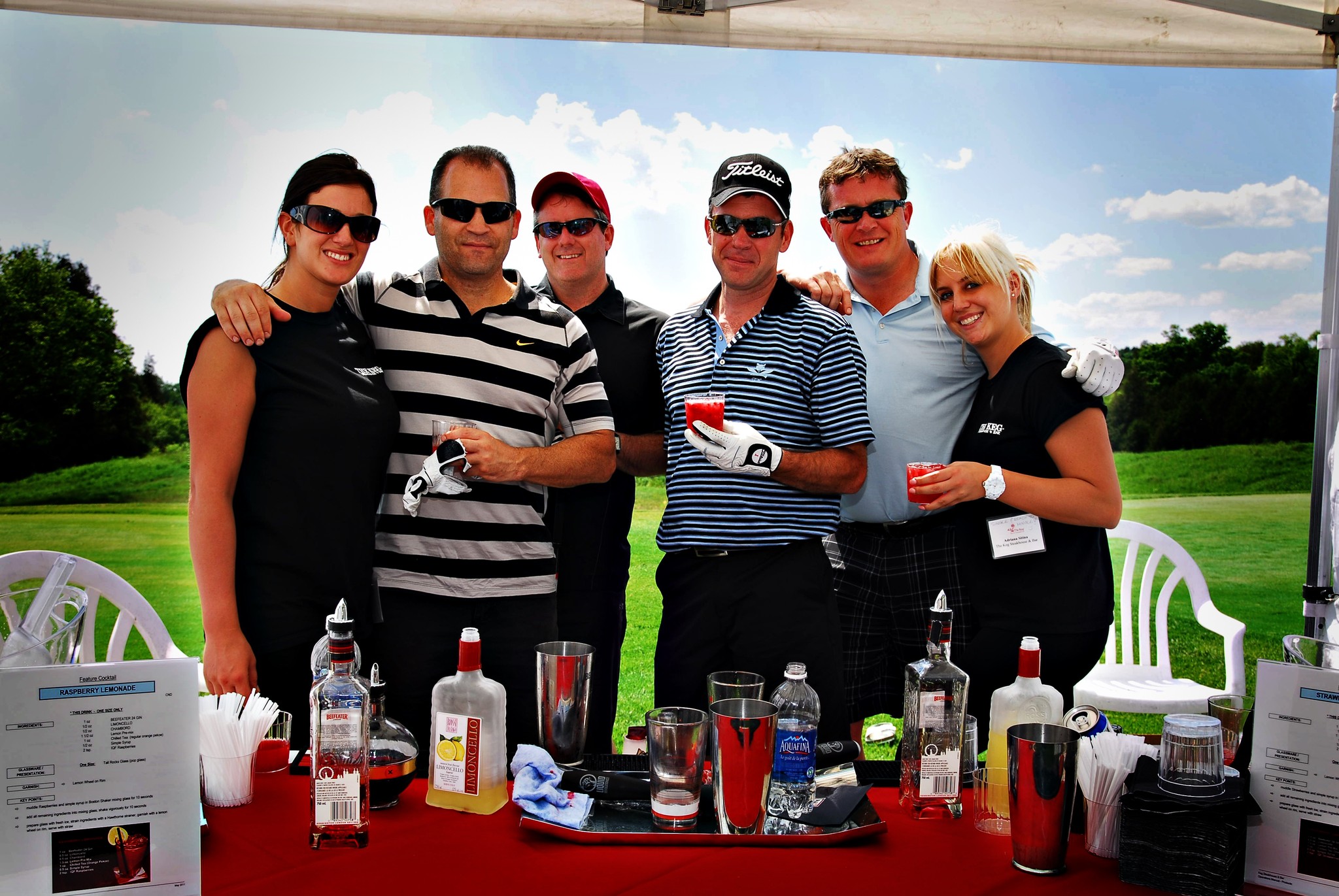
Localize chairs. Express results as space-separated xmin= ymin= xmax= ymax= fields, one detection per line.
xmin=0 ymin=545 xmax=192 ymax=666
xmin=1073 ymin=518 xmax=1248 ymax=731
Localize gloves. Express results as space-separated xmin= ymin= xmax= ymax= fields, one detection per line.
xmin=684 ymin=420 xmax=784 ymax=476
xmin=1061 ymin=337 xmax=1124 ymax=396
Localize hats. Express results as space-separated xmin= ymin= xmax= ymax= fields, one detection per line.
xmin=532 ymin=172 xmax=610 ymax=223
xmin=711 ymin=153 xmax=791 ymax=218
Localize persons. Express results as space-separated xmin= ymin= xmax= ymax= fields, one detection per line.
xmin=787 ymin=147 xmax=1124 ymax=723
xmin=180 ymin=151 xmax=401 ymax=750
xmin=212 ymin=144 xmax=617 ymax=779
xmin=525 ymin=171 xmax=672 ymax=754
xmin=655 ymin=153 xmax=877 ymax=741
xmin=909 ymin=229 xmax=1122 ymax=755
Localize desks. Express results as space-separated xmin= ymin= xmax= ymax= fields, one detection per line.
xmin=199 ymin=741 xmax=1298 ymax=896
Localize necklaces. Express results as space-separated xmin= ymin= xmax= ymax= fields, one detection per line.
xmin=1021 ymin=334 xmax=1032 ymax=344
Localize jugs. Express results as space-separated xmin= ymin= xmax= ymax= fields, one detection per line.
xmin=0 ymin=585 xmax=87 ymax=668
xmin=1282 ymin=635 xmax=1339 ymax=670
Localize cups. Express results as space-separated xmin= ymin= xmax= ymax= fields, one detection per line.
xmin=1082 ymin=796 xmax=1121 ymax=860
xmin=1157 ymin=713 xmax=1226 ymax=797
xmin=1208 ymin=695 xmax=1255 ymax=766
xmin=200 ymin=748 xmax=258 ymax=808
xmin=1006 ymin=723 xmax=1081 ymax=875
xmin=707 ymin=671 xmax=766 ymax=706
xmin=708 ymin=698 xmax=780 ymax=834
xmin=431 ymin=419 xmax=477 ymax=454
xmin=253 ymin=711 xmax=292 ymax=774
xmin=906 ymin=462 xmax=947 ymax=504
xmin=645 ymin=707 xmax=709 ymax=830
xmin=684 ymin=393 xmax=725 ymax=438
xmin=115 ymin=835 xmax=146 ymax=878
xmin=972 ymin=768 xmax=1012 ymax=836
xmin=1221 ymin=727 xmax=1238 ymax=765
xmin=962 ymin=714 xmax=978 ymax=783
xmin=533 ymin=642 xmax=596 ymax=766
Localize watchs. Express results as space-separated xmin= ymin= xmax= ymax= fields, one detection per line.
xmin=614 ymin=433 xmax=621 ymax=455
xmin=982 ymin=464 xmax=1006 ymax=500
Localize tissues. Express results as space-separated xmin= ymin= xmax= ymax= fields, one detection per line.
xmin=815 ymin=762 xmax=857 ymax=787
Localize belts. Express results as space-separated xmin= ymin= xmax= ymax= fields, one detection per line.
xmin=691 ymin=547 xmax=744 ymax=557
xmin=843 ymin=510 xmax=952 ymax=536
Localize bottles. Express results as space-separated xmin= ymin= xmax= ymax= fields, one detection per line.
xmin=558 ymin=770 xmax=651 ymax=800
xmin=767 ymin=662 xmax=821 ymax=819
xmin=897 ymin=589 xmax=970 ymax=819
xmin=366 ymin=663 xmax=420 ymax=809
xmin=310 ymin=614 xmax=361 ymax=681
xmin=816 ymin=740 xmax=861 ymax=770
xmin=308 ymin=598 xmax=370 ymax=849
xmin=985 ymin=636 xmax=1064 ymax=819
xmin=426 ymin=627 xmax=509 ymax=815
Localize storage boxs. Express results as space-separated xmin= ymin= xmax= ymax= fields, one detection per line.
xmin=0 ymin=656 xmax=193 ymax=896
xmin=1237 ymin=650 xmax=1339 ymax=896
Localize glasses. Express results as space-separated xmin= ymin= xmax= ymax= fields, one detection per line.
xmin=707 ymin=213 xmax=788 ymax=238
xmin=826 ymin=199 xmax=904 ymax=224
xmin=533 ymin=217 xmax=610 ymax=240
xmin=430 ymin=198 xmax=516 ymax=225
xmin=287 ymin=204 xmax=381 ymax=242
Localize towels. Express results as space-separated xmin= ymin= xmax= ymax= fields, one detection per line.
xmin=510 ymin=744 xmax=594 ymax=830
